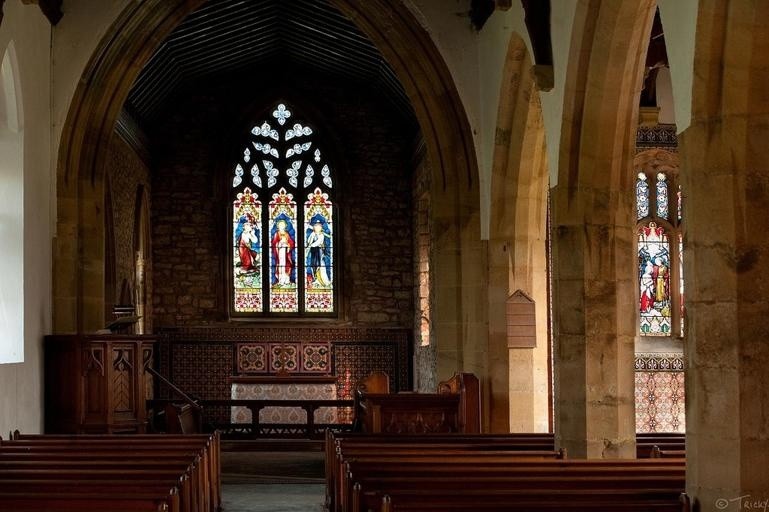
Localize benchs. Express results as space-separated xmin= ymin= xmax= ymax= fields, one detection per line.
xmin=323 ymin=424 xmax=685 ymax=511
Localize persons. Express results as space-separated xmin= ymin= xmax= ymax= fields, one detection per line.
xmin=237 ymin=217 xmax=258 ymax=274
xmin=272 ymin=220 xmax=294 ymax=284
xmin=640 ymin=257 xmax=669 ymax=313
xmin=307 ymin=220 xmax=330 ymax=286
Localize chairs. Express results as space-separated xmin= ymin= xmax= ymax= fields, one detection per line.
xmin=1 ymin=427 xmax=222 ymax=508
